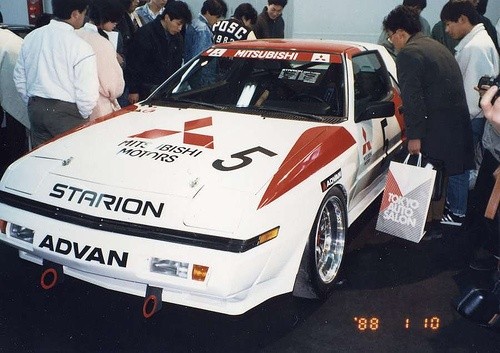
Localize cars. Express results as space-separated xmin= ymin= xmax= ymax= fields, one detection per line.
xmin=0 ymin=36 xmax=408 ymax=322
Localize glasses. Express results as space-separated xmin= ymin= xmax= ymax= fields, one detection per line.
xmin=387 ymin=32 xmax=394 ymax=43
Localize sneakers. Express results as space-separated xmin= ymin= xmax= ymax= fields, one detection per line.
xmin=439 ymin=213 xmax=464 ymax=226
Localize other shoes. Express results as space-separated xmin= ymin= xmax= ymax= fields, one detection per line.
xmin=470 ymin=256 xmax=498 ymax=271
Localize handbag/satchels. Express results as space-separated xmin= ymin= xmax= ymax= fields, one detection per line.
xmin=375 ymin=150 xmax=437 ymax=244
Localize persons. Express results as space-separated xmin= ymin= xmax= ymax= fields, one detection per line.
xmin=378 ymin=0 xmax=500 ymax=327
xmin=0 ymin=0 xmax=287 ymax=180
